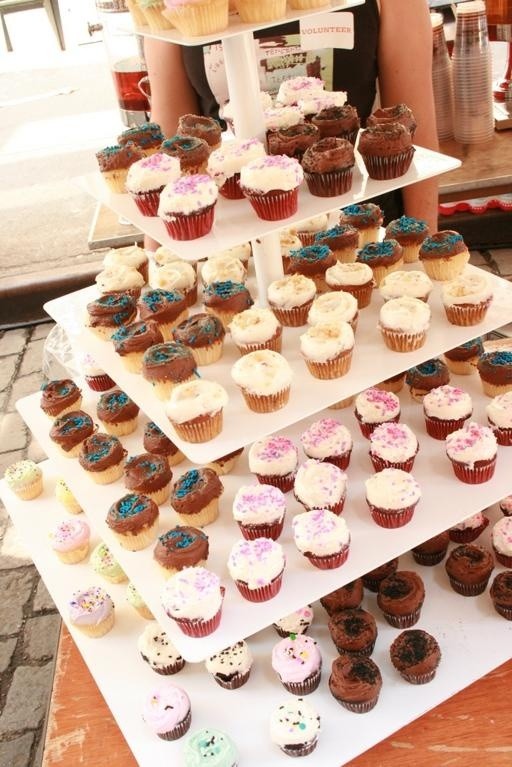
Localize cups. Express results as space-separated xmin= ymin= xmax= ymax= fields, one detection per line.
xmin=424 ymin=1 xmax=498 ymax=144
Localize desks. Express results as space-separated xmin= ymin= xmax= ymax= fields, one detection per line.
xmin=88 ymin=126 xmax=512 ymax=249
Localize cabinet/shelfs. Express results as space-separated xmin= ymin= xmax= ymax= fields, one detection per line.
xmin=0 ymin=0 xmax=512 ymax=767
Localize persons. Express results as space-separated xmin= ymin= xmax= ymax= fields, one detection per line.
xmin=143 ymin=0 xmax=439 ymax=237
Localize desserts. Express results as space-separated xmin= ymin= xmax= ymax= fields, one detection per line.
xmin=4 ymin=1 xmax=512 ymax=767
